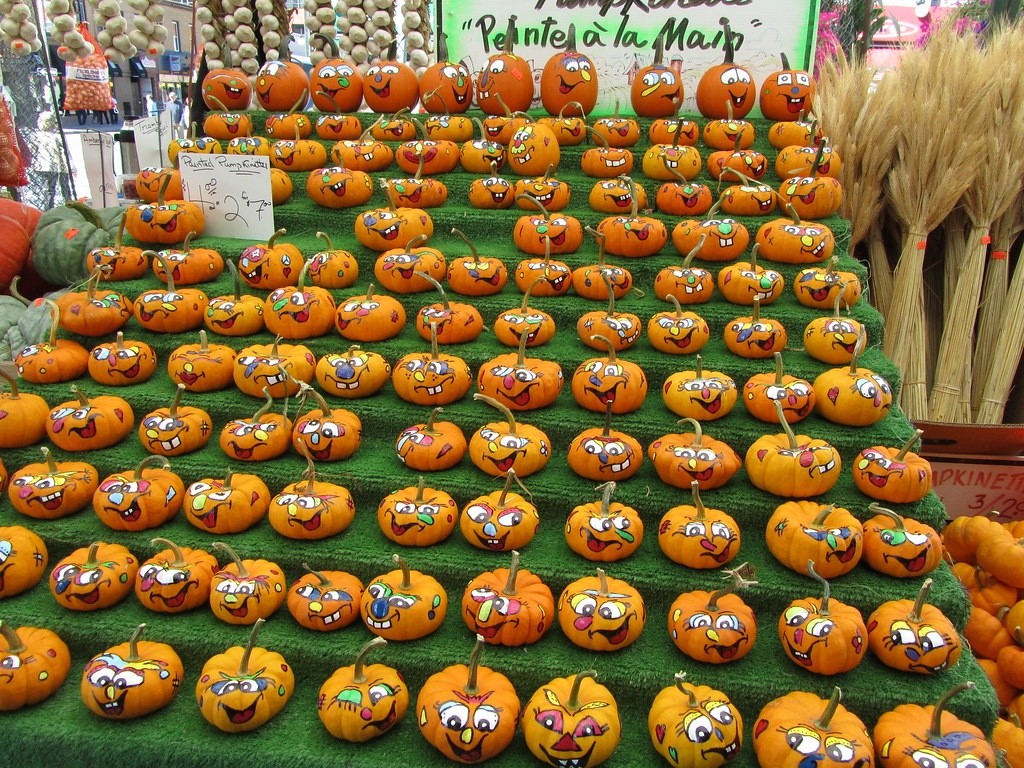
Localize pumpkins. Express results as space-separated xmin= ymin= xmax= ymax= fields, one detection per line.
xmin=942 ymin=515 xmax=1024 ymax=768
xmin=0 ymin=197 xmax=139 ymax=363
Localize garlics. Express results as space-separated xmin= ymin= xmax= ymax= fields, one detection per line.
xmin=0 ymin=0 xmax=435 ymax=189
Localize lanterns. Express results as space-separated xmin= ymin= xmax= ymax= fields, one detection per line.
xmin=667 ymin=562 xmax=758 ymax=666
xmin=416 ymin=634 xmax=523 ymax=763
xmin=646 ymin=669 xmax=746 ymax=768
xmin=0 ymin=620 xmax=71 ymax=714
xmin=557 ymin=567 xmax=650 ymax=657
xmin=315 ymin=633 xmax=412 ymax=747
xmin=751 ymin=683 xmax=876 ymax=768
xmin=0 ymin=23 xmax=950 ymax=578
xmin=462 ymin=549 xmax=560 ymax=646
xmin=135 ymin=534 xmax=219 ymax=616
xmin=778 ymin=557 xmax=870 ymax=679
xmin=869 ymin=681 xmax=998 ymax=768
xmin=867 ymin=578 xmax=965 ymax=677
xmin=360 ymin=548 xmax=450 ymax=644
xmin=207 ymin=538 xmax=287 ymax=628
xmin=48 ymin=537 xmax=140 ymax=617
xmin=285 ymin=562 xmax=367 ymax=636
xmin=523 ymin=664 xmax=623 ymax=767
xmin=193 ymin=617 xmax=296 ymax=736
xmin=79 ymin=620 xmax=186 ymax=720
